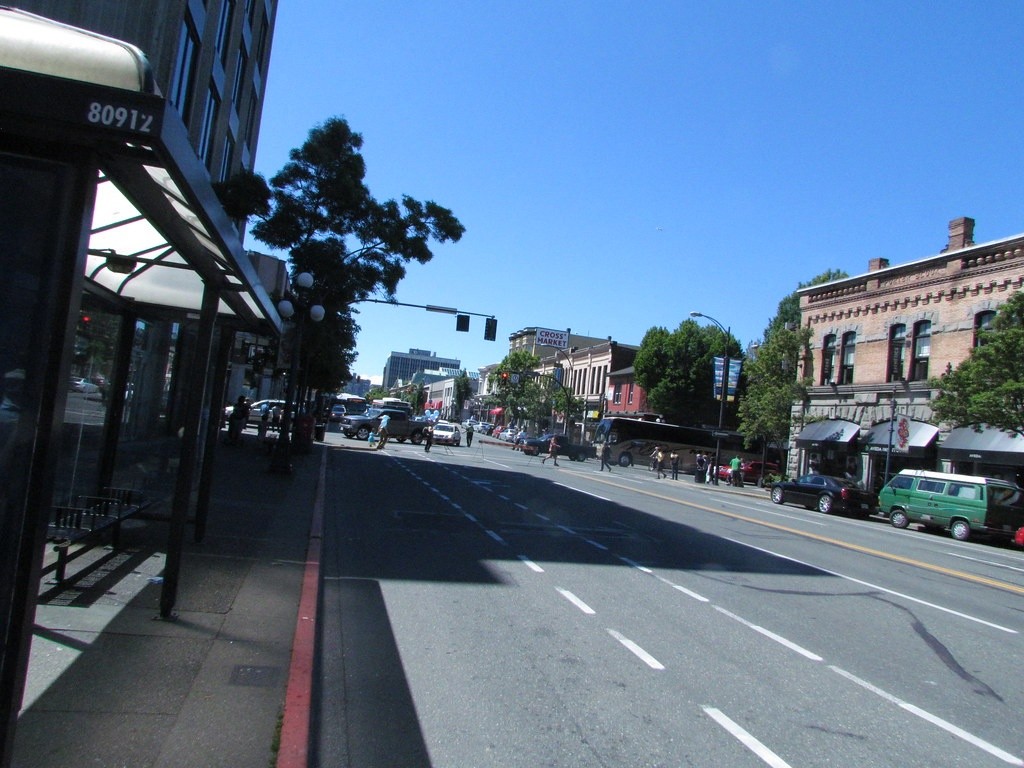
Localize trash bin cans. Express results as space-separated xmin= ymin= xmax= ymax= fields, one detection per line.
xmin=695 ymin=467 xmax=706 ymax=484
xmin=290 ymin=415 xmax=316 ymax=454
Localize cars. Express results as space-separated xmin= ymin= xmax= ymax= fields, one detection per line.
xmin=426 ymin=424 xmax=462 ymax=446
xmin=67 ymin=373 xmax=99 ymax=394
xmin=513 ymin=431 xmax=527 ymax=445
xmin=498 ymin=428 xmax=518 ymax=443
xmin=491 ymin=425 xmax=508 ymax=439
xmin=462 ymin=419 xmax=498 ymax=435
xmin=328 ymin=404 xmax=347 ymax=423
xmin=769 ymin=474 xmax=877 ymax=516
xmin=121 ymin=382 xmax=135 ymax=404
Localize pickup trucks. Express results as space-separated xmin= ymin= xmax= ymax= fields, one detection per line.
xmin=518 ymin=433 xmax=597 ymax=463
xmin=338 ymin=408 xmax=431 ymax=445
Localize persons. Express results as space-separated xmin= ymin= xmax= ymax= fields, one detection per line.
xmin=600 ymin=441 xmax=612 ymax=472
xmin=230 ymin=396 xmax=282 ymax=442
xmin=670 ymin=450 xmax=679 ymax=480
xmin=425 ymin=425 xmax=434 ymax=452
xmin=695 ymin=453 xmax=714 ymax=484
xmin=367 ymin=415 xmax=391 ymax=449
xmin=650 ymin=447 xmax=666 ymax=479
xmin=543 ymin=433 xmax=560 ymax=466
xmin=726 ymin=455 xmax=745 ymax=488
xmin=512 ymin=427 xmax=527 ymax=451
xmin=465 ymin=423 xmax=474 ymax=447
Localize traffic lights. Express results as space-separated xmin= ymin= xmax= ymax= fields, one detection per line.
xmin=79 ymin=314 xmax=93 ymax=326
xmin=502 ymin=371 xmax=509 ymax=388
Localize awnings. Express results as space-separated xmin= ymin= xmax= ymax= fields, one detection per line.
xmin=858 ymin=416 xmax=940 ymax=458
xmin=489 ymin=407 xmax=503 ymax=415
xmin=936 ymin=421 xmax=1024 ymax=466
xmin=796 ymin=419 xmax=860 ymax=452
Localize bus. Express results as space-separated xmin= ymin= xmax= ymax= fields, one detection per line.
xmin=335 ymin=392 xmax=367 ymax=415
xmin=592 ymin=416 xmax=764 ymax=475
xmin=382 ymin=397 xmax=414 ymax=419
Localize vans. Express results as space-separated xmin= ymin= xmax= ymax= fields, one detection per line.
xmin=366 ymin=399 xmax=383 ymax=410
xmin=877 ymin=468 xmax=1024 ymax=541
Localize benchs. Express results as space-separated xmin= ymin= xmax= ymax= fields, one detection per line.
xmin=46 ymin=487 xmax=154 ymax=584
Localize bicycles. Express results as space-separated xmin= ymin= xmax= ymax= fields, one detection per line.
xmin=648 ymin=455 xmax=660 ymax=471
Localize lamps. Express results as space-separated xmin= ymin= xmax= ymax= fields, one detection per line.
xmin=106 ymin=253 xmax=136 ymax=274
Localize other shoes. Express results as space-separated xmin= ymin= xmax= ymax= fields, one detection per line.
xmin=542 ymin=459 xmax=545 ymax=464
xmin=664 ymin=475 xmax=667 ymax=478
xmin=377 ymin=445 xmax=385 ymax=449
xmin=609 ymin=468 xmax=611 ymax=472
xmin=425 ymin=449 xmax=430 ymax=452
xmin=554 ymin=463 xmax=559 ymax=466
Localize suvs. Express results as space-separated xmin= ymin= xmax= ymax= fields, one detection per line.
xmin=718 ymin=460 xmax=780 ymax=485
xmin=225 ymin=398 xmax=304 ymax=425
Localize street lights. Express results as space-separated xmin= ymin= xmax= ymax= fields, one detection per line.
xmin=540 ymin=343 xmax=574 ymax=435
xmin=689 ymin=310 xmax=731 ymax=485
xmin=265 ymin=272 xmax=326 ymax=474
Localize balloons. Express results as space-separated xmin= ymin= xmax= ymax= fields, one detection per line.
xmin=425 ymin=409 xmax=439 ymax=420
